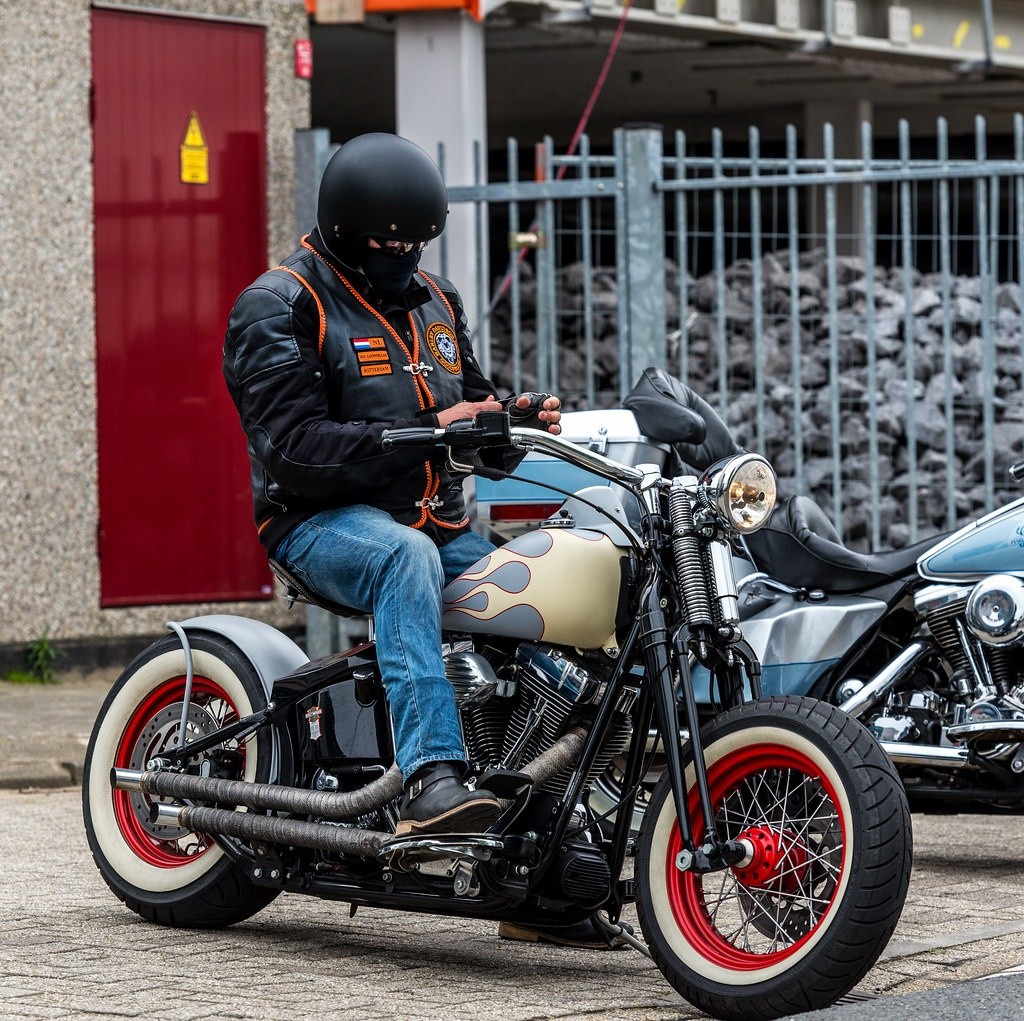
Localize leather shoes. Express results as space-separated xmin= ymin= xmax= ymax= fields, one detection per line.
xmin=396 ymin=759 xmax=503 ymax=835
xmin=498 ymin=906 xmax=633 ymax=948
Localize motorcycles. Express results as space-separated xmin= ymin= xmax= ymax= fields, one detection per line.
xmin=495 ymin=405 xmax=1024 ymax=823
xmin=77 ymin=363 xmax=918 ymax=1021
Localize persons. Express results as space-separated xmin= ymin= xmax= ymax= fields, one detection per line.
xmin=221 ymin=134 xmax=634 ymax=953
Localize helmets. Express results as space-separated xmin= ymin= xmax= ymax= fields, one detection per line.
xmin=315 ymin=132 xmax=448 ymax=295
